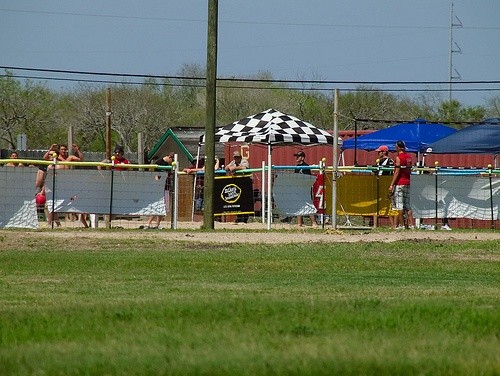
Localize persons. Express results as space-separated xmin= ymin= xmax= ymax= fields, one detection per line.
xmin=98 ymin=146 xmax=130 ymax=171
xmin=36 ymin=143 xmax=88 ymax=227
xmin=225 ymin=151 xmax=253 ymax=223
xmin=390 ymin=141 xmax=415 ymax=228
xmin=144 ymin=154 xmax=174 ymax=228
xmin=294 ymin=151 xmax=317 ymax=227
xmin=372 ymin=145 xmax=394 ymax=228
xmin=441 ymin=217 xmax=452 ymax=230
xmin=5 ymin=152 xmax=23 ymax=167
xmin=184 ymin=159 xmax=222 ymax=222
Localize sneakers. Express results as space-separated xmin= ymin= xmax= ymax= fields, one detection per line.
xmin=440 ymin=224 xmax=453 ymax=230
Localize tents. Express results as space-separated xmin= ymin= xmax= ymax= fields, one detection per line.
xmin=418 ymin=118 xmax=500 ymax=229
xmin=334 ymin=119 xmax=458 ymax=227
xmin=192 ymin=105 xmax=345 ymax=223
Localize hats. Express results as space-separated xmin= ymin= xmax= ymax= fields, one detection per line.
xmin=294 ymin=151 xmax=305 ymax=158
xmin=171 ymin=149 xmax=180 ymax=158
xmin=232 ymin=150 xmax=241 ymax=158
xmin=396 ymin=141 xmax=406 ymax=149
xmin=375 ymin=145 xmax=389 ymax=152
xmin=114 ymin=146 xmax=124 ymax=152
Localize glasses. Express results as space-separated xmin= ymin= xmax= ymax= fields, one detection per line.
xmin=48 ymin=154 xmax=53 ymax=156
